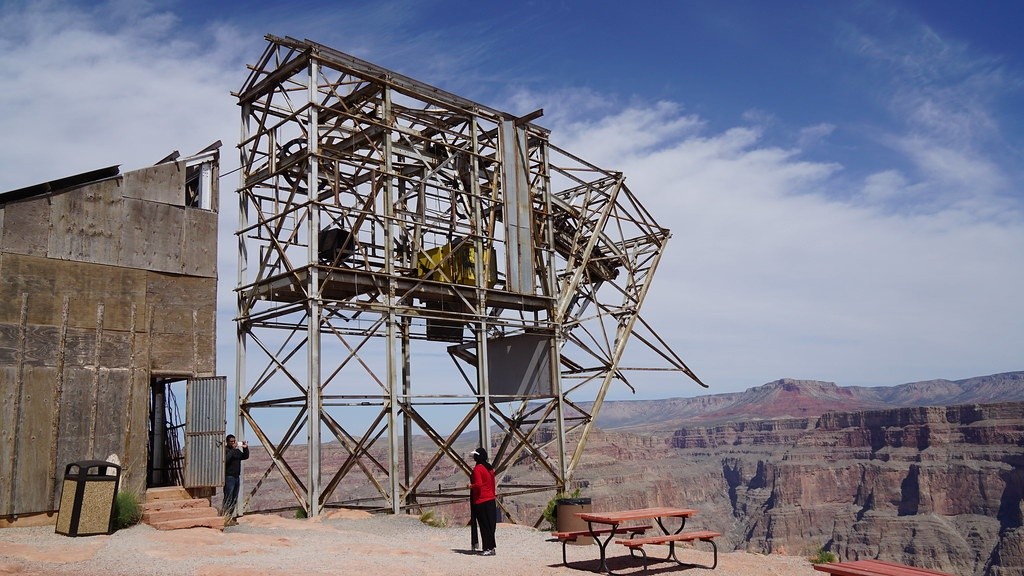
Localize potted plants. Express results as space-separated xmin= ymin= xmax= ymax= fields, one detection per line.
xmin=543 ymin=489 xmax=593 ymax=545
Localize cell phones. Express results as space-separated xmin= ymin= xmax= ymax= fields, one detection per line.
xmin=236 ymin=442 xmax=243 ymax=446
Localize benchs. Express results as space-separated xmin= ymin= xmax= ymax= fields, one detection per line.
xmin=552 ymin=525 xmax=653 ymax=569
xmin=814 ymin=559 xmax=956 ymax=576
xmin=615 ymin=531 xmax=721 ymax=576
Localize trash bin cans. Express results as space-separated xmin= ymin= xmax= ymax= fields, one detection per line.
xmin=54 ymin=460 xmax=122 ymax=537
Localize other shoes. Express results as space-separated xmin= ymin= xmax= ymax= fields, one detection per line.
xmin=228 ymin=517 xmax=239 ymax=526
xmin=479 ymin=547 xmax=496 ymax=556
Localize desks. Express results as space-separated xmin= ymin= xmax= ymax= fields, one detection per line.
xmin=574 ymin=507 xmax=699 ymax=576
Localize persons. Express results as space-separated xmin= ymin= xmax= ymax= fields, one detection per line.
xmin=466 ymin=448 xmax=497 ymax=556
xmin=220 ymin=434 xmax=250 ymax=526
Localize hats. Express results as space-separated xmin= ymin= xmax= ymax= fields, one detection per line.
xmin=471 ymin=450 xmax=480 ymax=456
